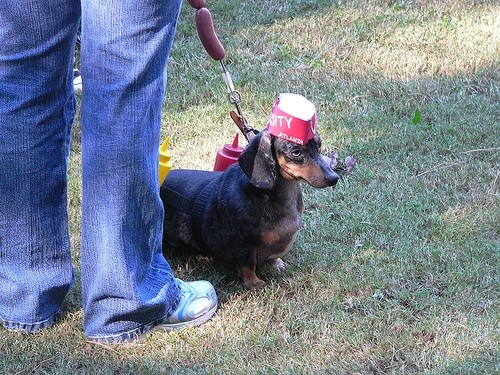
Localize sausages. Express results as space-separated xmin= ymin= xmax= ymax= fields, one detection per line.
xmin=188 ymin=0 xmax=224 ymax=61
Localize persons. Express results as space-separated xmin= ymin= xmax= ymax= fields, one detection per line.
xmin=0 ymin=0 xmax=218 ymax=344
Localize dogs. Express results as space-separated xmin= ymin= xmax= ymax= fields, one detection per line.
xmin=161 ymin=93 xmax=341 ymax=292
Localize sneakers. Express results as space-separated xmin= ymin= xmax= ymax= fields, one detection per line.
xmin=155 ymin=279 xmax=218 ymax=329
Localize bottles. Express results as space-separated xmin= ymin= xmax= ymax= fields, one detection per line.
xmin=158 ymin=132 xmax=174 ymax=188
xmin=211 ymin=133 xmax=243 ymax=171
